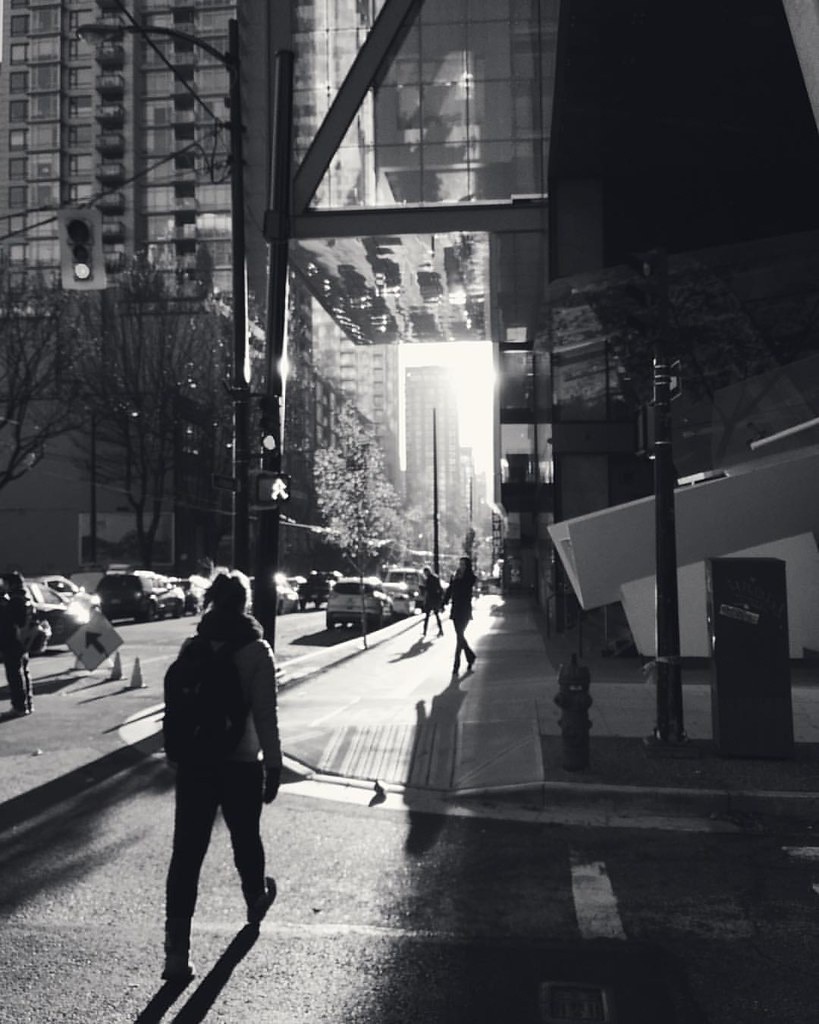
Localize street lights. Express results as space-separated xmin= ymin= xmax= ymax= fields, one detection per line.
xmin=78 ymin=21 xmax=248 ymax=578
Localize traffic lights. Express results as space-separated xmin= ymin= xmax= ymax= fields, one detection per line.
xmin=258 ymin=396 xmax=280 ymax=457
xmin=254 ymin=474 xmax=291 ymax=503
xmin=58 ymin=208 xmax=108 ymax=292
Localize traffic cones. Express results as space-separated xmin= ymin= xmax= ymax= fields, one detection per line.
xmin=106 ymin=652 xmax=128 ymax=682
xmin=124 ymin=657 xmax=149 ymax=690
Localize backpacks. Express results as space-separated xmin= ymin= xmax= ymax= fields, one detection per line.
xmin=10 ymin=595 xmax=52 ymax=654
xmin=165 ymin=636 xmax=256 ymax=754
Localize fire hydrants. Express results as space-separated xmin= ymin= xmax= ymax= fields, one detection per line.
xmin=557 ymin=657 xmax=595 ymax=763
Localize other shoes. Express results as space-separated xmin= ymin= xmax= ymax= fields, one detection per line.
xmin=247 ymin=877 xmax=277 ymax=923
xmin=10 ymin=706 xmax=33 ymax=715
xmin=453 ymin=664 xmax=460 ymax=674
xmin=161 ymin=961 xmax=195 ymax=980
xmin=468 ymin=655 xmax=476 ymax=670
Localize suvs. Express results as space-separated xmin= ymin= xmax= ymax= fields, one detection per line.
xmin=325 ymin=578 xmax=391 ymax=627
xmin=299 ymin=571 xmax=342 ymax=608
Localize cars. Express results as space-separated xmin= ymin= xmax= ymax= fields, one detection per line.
xmin=387 ymin=583 xmax=416 ymax=619
xmin=15 ymin=583 xmax=78 ymax=651
xmin=29 ymin=576 xmax=105 ymax=625
xmin=94 ymin=568 xmax=186 ymax=621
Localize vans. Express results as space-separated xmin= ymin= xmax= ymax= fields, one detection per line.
xmin=382 ymin=568 xmax=426 ymax=608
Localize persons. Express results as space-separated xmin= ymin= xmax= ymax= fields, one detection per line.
xmin=417 ymin=565 xmax=446 ymax=637
xmin=0 ymin=571 xmax=35 ymax=715
xmin=440 ymin=557 xmax=477 ymax=675
xmin=160 ymin=567 xmax=282 ymax=984
